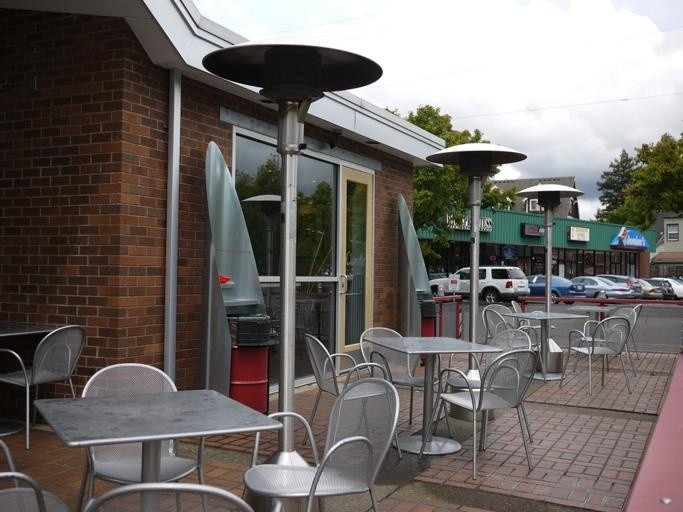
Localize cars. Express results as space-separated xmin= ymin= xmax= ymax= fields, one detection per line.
xmin=428 ymin=265 xmax=682 ymax=306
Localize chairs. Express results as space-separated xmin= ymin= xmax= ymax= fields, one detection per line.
xmin=302 ymin=292 xmax=646 ymax=482
xmin=1 ymin=322 xmax=400 ymax=511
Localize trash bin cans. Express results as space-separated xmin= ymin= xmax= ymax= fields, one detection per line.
xmin=226 ymin=313 xmax=271 ymax=415
xmin=418 ymin=299 xmax=437 ymax=367
